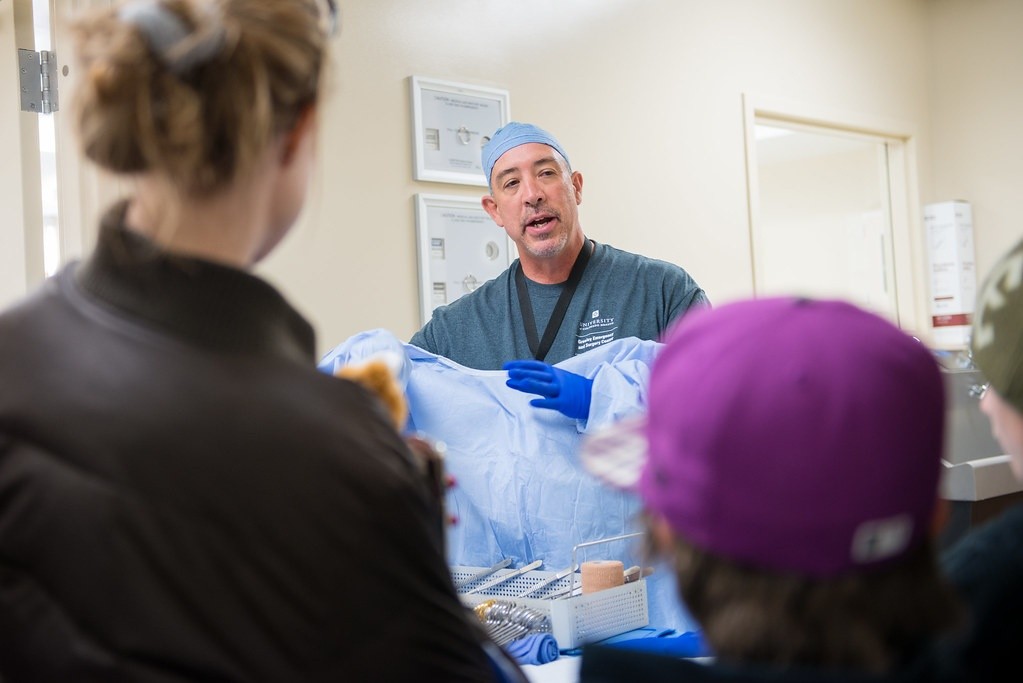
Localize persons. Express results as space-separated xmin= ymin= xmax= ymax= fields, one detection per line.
xmin=641 ymin=295 xmax=965 ymax=683
xmin=3 ymin=0 xmax=529 ymax=683
xmin=409 ymin=121 xmax=714 ymax=475
xmin=938 ymin=241 xmax=1023 ymax=607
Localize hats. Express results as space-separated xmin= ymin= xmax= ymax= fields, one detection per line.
xmin=970 ymin=237 xmax=1023 ymax=414
xmin=641 ymin=294 xmax=944 ymax=576
xmin=481 ymin=122 xmax=571 ymax=198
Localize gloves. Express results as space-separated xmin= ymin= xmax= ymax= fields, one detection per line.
xmin=502 ymin=359 xmax=593 ymax=419
